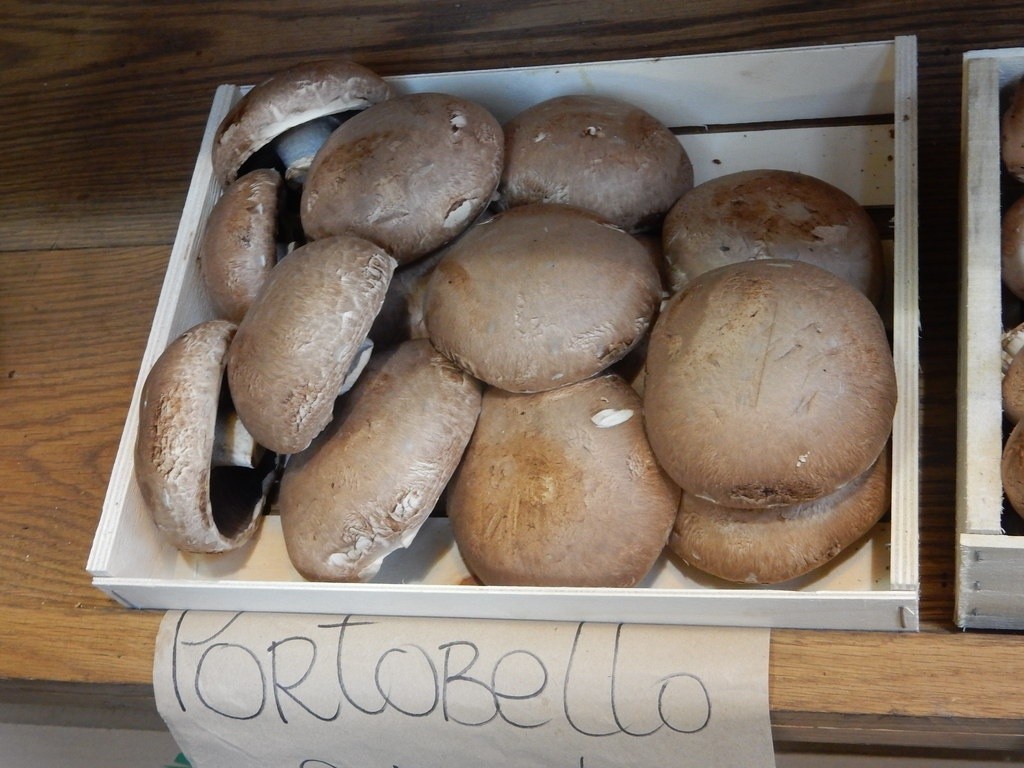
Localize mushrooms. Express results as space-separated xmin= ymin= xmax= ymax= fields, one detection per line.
xmin=997 ymin=70 xmax=1024 ymax=533
xmin=130 ymin=55 xmax=898 ymax=590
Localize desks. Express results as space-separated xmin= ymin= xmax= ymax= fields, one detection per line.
xmin=2 ymin=0 xmax=1024 ymax=768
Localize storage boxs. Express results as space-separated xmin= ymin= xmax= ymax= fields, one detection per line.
xmin=87 ymin=34 xmax=922 ymax=632
xmin=949 ymin=46 xmax=1023 ymax=631
